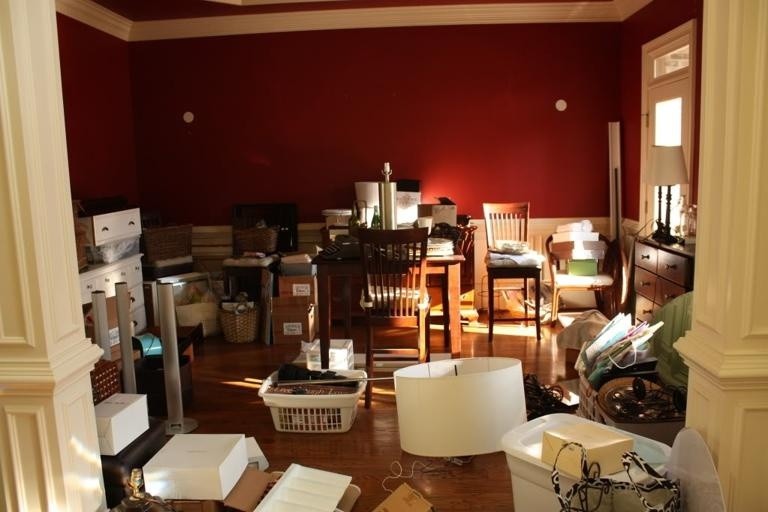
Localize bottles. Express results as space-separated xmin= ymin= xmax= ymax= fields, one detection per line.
xmin=371 ymin=207 xmax=382 ymax=229
xmin=680 ymin=204 xmax=696 ymax=237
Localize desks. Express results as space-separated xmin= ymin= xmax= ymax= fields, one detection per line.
xmin=311 ymin=245 xmax=465 ymax=369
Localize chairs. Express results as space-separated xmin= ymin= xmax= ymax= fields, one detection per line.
xmin=482 ymin=202 xmax=541 ymax=340
xmin=357 ymin=227 xmax=431 ymax=408
xmin=545 ymin=234 xmax=618 ymax=327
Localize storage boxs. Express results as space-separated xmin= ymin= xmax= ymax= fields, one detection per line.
xmin=502 ymin=413 xmax=673 ymax=511
xmin=552 ymin=223 xmax=598 ymax=276
xmin=566 ymin=348 xmax=579 ymax=379
xmin=542 ymin=424 xmax=633 ymax=480
xmin=79 ymin=199 xmax=356 ymax=512
xmin=418 ymin=197 xmax=456 ymax=229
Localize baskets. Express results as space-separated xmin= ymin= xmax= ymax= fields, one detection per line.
xmin=576 ymin=374 xmax=599 ymax=421
xmin=219 ymin=294 xmax=260 ymax=344
xmin=141 ymin=214 xmax=193 ymax=262
xmin=90 ymin=360 xmax=121 ymax=406
xmin=257 ymin=369 xmax=368 ymax=433
xmin=234 ymin=225 xmax=278 ymax=255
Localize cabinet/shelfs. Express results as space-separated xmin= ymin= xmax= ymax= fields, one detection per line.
xmin=635 ymin=233 xmax=695 ymax=325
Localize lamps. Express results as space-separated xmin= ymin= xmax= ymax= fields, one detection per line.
xmin=393 ymin=357 xmax=527 ymax=466
xmin=647 ymin=145 xmax=689 ymax=245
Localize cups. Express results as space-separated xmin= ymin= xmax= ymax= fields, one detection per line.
xmin=417 ymin=217 xmax=434 ymax=236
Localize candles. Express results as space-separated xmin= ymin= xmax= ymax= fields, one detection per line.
xmin=384 ymin=163 xmax=390 ymax=172
